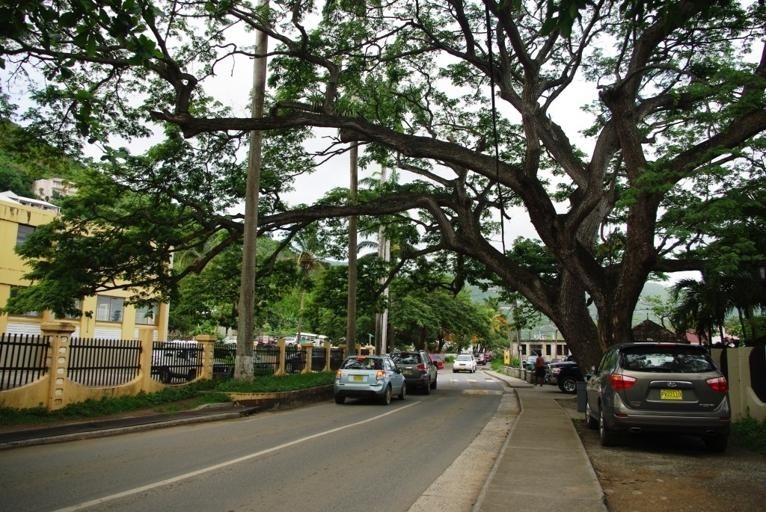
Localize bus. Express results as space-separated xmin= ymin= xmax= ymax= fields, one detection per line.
xmin=296 ymin=331 xmax=330 ymax=346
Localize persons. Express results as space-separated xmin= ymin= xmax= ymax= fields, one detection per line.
xmin=534 ymin=352 xmax=546 ymax=387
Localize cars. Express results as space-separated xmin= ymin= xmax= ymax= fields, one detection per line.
xmin=225 ymin=332 xmax=295 ymax=346
xmin=522 ymin=354 xmax=550 ymax=369
xmin=429 ymin=354 xmax=444 ymax=369
xmin=214 ymin=346 xmax=275 ymax=376
xmin=485 ymin=353 xmax=492 ymax=362
xmin=452 ymin=354 xmax=477 ymax=373
xmin=284 ymin=347 xmax=344 ymax=373
xmin=474 ymin=352 xmax=486 ymax=365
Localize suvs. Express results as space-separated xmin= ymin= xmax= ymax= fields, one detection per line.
xmin=587 ymin=342 xmax=730 ymax=454
xmin=548 ymin=353 xmax=584 ymax=394
xmin=156 ymin=340 xmax=227 ymax=383
xmin=333 ymin=355 xmax=407 ymax=405
xmin=389 ymin=350 xmax=438 ymax=394
